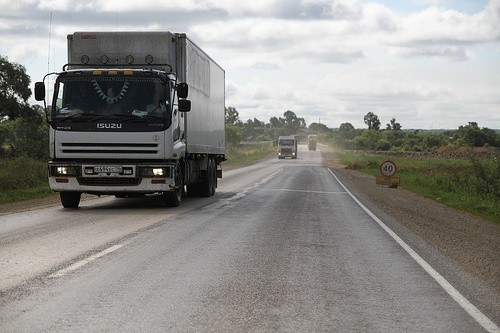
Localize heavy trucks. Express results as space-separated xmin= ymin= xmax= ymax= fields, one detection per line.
xmin=34 ymin=33 xmax=227 ymax=207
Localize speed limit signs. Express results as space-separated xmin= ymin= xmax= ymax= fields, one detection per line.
xmin=380 ymin=161 xmax=397 ymax=177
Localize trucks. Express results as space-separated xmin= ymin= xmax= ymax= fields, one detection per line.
xmin=274 ymin=135 xmax=298 ymax=159
xmin=308 ymin=134 xmax=317 ymax=151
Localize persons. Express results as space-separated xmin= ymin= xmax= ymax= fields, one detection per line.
xmin=64 ymin=82 xmax=98 ymax=113
xmin=145 ymin=91 xmax=168 ymax=114
